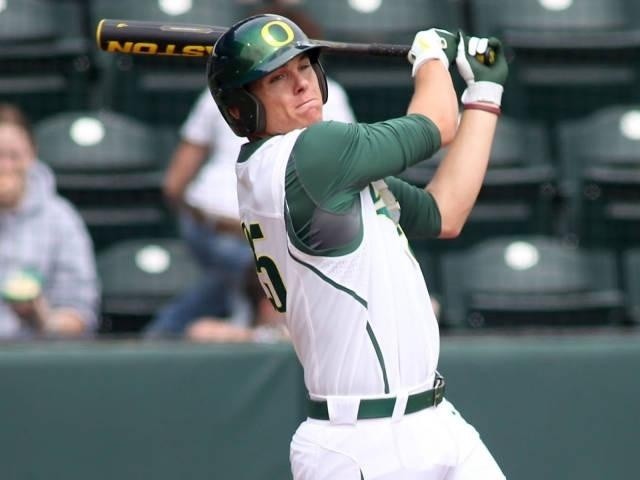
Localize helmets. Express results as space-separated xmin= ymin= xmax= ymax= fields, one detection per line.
xmin=205 ymin=12 xmax=328 ymax=136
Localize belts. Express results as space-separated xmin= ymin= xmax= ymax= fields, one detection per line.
xmin=183 ymin=201 xmax=246 ymax=242
xmin=306 ymin=369 xmax=446 ymax=422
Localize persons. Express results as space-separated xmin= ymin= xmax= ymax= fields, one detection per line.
xmin=206 ymin=12 xmax=509 ymax=480
xmin=143 ymin=8 xmax=359 ymax=341
xmin=0 ymin=104 xmax=103 ymax=340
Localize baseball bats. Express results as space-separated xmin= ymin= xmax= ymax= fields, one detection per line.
xmin=96 ymin=18 xmax=497 ymax=67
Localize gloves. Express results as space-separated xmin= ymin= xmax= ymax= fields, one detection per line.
xmin=406 ymin=28 xmax=457 ymax=76
xmin=455 ymin=28 xmax=509 ymax=117
